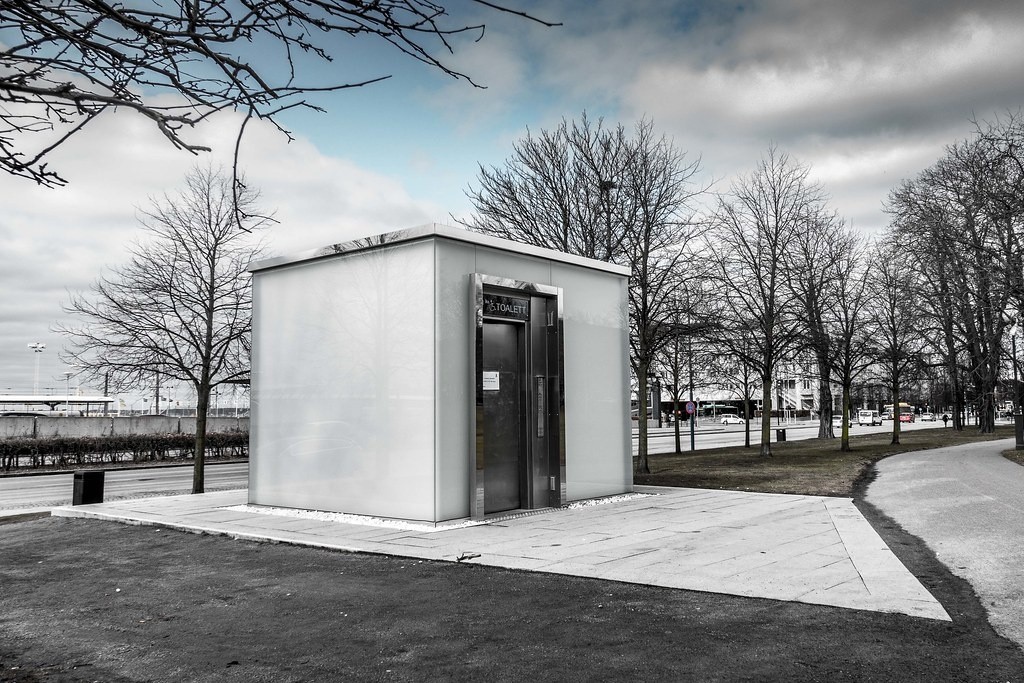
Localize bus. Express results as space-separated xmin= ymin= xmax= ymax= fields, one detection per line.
xmin=882 ymin=403 xmax=912 ymax=418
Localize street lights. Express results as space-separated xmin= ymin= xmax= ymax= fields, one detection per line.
xmin=27 ymin=339 xmax=45 ymax=396
xmin=63 ymin=372 xmax=75 ymax=418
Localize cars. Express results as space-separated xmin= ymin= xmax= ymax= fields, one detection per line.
xmin=720 ymin=413 xmax=745 ymax=425
xmin=900 ymin=412 xmax=915 ymax=423
xmin=921 ymin=413 xmax=937 ymax=422
xmin=942 ymin=410 xmax=953 ymax=420
xmin=882 ymin=412 xmax=892 ymax=420
xmin=832 ymin=415 xmax=852 ymax=428
xmin=663 ymin=414 xmax=675 ymax=421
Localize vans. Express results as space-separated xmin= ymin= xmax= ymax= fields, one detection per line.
xmin=859 ymin=410 xmax=883 ymax=426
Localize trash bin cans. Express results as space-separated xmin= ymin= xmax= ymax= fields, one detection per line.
xmin=776 ymin=429 xmax=786 ymax=441
xmin=73 ymin=469 xmax=105 ymax=505
